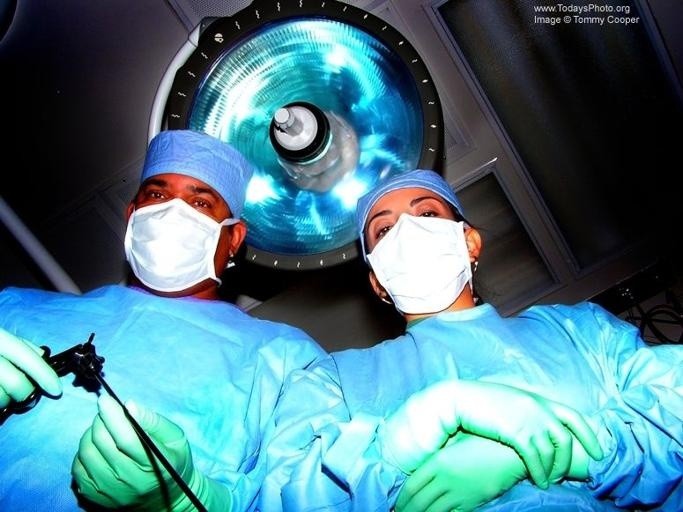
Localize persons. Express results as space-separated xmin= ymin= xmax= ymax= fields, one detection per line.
xmin=261 ymin=169 xmax=683 ymax=511
xmin=1 ymin=126 xmax=329 ymax=512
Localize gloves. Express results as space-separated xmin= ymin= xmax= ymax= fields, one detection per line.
xmin=375 ymin=381 xmax=603 ymax=488
xmin=393 ymin=435 xmax=590 ymax=512
xmin=71 ymin=396 xmax=233 ymax=512
xmin=0 ymin=326 xmax=63 ymax=406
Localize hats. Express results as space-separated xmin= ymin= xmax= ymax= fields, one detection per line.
xmin=142 ymin=129 xmax=253 ymax=218
xmin=357 ymin=167 xmax=466 ymax=267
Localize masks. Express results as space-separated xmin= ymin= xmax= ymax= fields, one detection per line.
xmin=367 ymin=214 xmax=470 ymax=314
xmin=124 ymin=201 xmax=240 ymax=292
